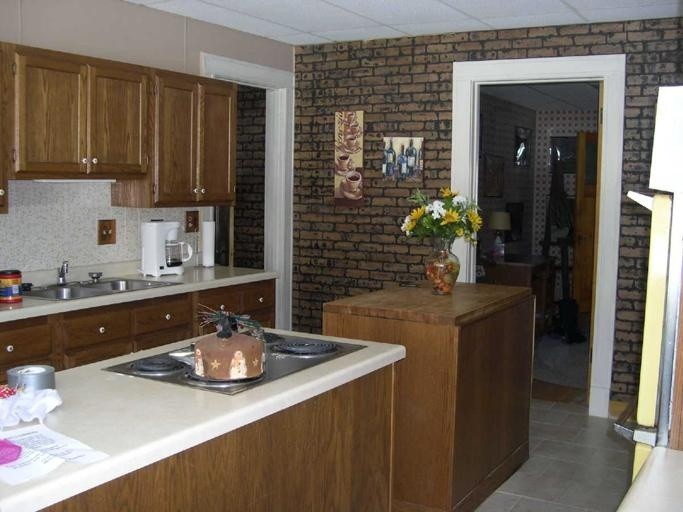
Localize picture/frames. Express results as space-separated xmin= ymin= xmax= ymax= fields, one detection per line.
xmin=479 ymin=114 xmax=483 ymax=158
xmin=483 ymin=153 xmax=505 ymax=198
xmin=512 ymin=126 xmax=532 ymax=168
xmin=505 ymin=202 xmax=524 ymax=242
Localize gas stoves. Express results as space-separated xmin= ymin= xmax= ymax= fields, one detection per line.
xmin=102 ymin=332 xmax=368 ymax=395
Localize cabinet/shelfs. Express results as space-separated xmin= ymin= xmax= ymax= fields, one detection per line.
xmin=59 ymin=301 xmax=134 ymax=371
xmin=196 ymin=284 xmax=241 ymax=338
xmin=241 ymin=278 xmax=276 ymax=331
xmin=323 ymin=280 xmax=535 ymax=512
xmin=134 ymin=292 xmax=196 ymax=354
xmin=481 ymin=253 xmax=554 ymax=336
xmin=0 ymin=313 xmax=59 ymax=384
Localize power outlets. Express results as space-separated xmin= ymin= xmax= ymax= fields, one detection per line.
xmin=185 ymin=211 xmax=199 ymax=233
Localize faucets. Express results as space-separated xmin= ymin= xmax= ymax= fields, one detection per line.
xmin=56 ymin=261 xmax=68 ymax=283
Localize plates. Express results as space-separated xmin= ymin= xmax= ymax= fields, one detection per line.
xmin=343 ymin=191 xmax=362 ymax=200
xmin=336 ymin=161 xmax=356 ymax=174
xmin=343 ymin=146 xmax=361 ymax=154
xmin=336 ymin=169 xmax=354 ymax=176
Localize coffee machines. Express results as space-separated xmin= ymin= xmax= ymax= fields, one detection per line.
xmin=141 ymin=219 xmax=192 ymax=277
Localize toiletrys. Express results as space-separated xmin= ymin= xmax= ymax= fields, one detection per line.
xmin=492 ymin=236 xmax=504 ymax=258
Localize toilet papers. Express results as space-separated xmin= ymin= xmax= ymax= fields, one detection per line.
xmin=202 ymin=221 xmax=215 ymax=267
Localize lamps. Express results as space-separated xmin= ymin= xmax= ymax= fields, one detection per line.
xmin=488 ymin=211 xmax=511 ymax=245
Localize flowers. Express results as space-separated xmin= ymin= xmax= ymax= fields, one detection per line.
xmin=400 ymin=188 xmax=483 ymax=246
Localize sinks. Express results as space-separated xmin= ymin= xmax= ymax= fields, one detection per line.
xmin=24 ymin=287 xmax=107 ymax=301
xmin=72 ymin=279 xmax=184 ymax=290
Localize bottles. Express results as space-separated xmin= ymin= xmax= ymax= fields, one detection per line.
xmin=418 ymin=138 xmax=423 ymax=171
xmin=406 ymin=139 xmax=417 ymax=176
xmin=397 ymin=145 xmax=406 ymax=180
xmin=382 ymin=142 xmax=387 ymax=176
xmin=387 ymin=139 xmax=396 ymax=178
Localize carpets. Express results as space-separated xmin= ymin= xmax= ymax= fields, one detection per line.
xmin=533 ymin=312 xmax=590 ymax=388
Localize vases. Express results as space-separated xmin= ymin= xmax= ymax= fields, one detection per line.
xmin=424 ymin=238 xmax=460 ymax=296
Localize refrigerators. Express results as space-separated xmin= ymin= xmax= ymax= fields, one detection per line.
xmin=617 ymin=85 xmax=682 ymax=487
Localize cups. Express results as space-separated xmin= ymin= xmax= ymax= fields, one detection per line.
xmin=337 ymin=154 xmax=350 ymax=170
xmin=344 ymin=135 xmax=357 ymax=147
xmin=345 ymin=111 xmax=355 ymax=122
xmin=350 ymin=121 xmax=360 ymax=134
xmin=341 ymin=172 xmax=361 ymax=191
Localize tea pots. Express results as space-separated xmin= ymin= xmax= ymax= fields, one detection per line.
xmin=192 ymin=308 xmax=264 ymax=380
